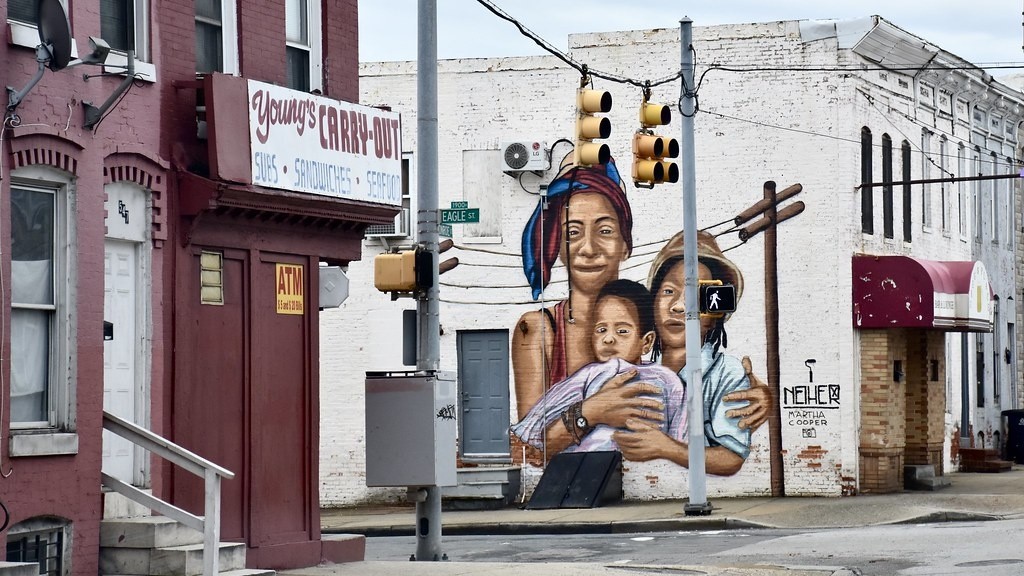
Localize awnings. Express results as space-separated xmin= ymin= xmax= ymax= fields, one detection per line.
xmin=851 ymin=256 xmax=995 ymax=333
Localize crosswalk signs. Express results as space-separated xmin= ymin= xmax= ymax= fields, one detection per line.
xmin=705 ymin=285 xmax=737 ymax=312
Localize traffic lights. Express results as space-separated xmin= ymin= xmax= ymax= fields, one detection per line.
xmin=574 ymin=88 xmax=612 ymax=170
xmin=631 ymin=101 xmax=679 ymax=185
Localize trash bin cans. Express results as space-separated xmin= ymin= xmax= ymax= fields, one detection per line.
xmin=1001 ymin=409 xmax=1024 ymax=466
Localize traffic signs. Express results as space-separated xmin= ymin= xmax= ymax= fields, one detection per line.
xmin=439 ymin=209 xmax=480 ymax=224
xmin=438 ymin=224 xmax=453 ymax=238
